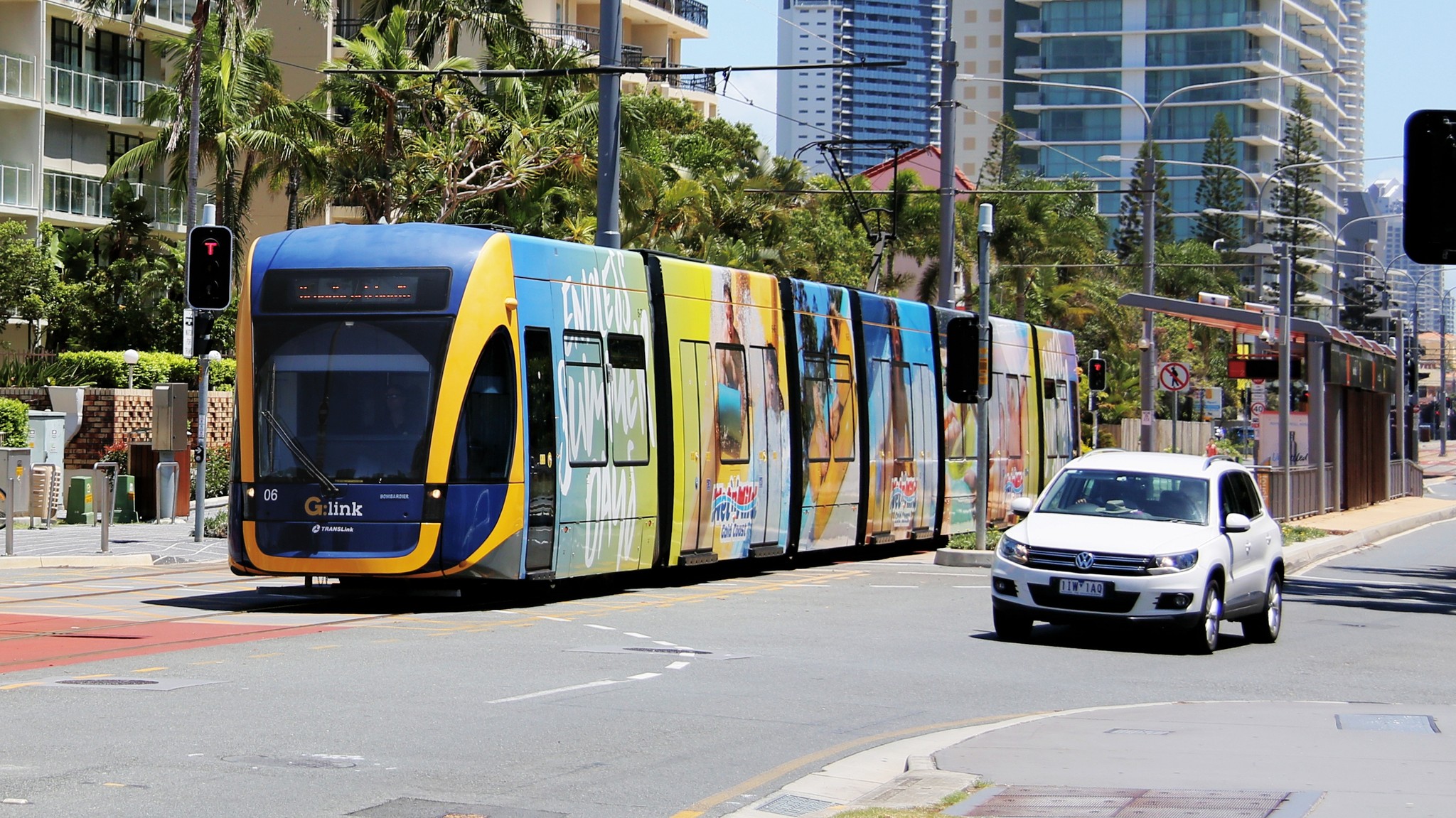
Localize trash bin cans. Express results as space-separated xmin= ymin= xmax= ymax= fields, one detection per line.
xmin=1419 ymin=424 xmax=1431 ymax=441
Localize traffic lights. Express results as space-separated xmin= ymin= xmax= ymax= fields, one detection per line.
xmin=187 ymin=224 xmax=234 ymax=312
xmin=1087 ymin=357 xmax=1107 ymax=392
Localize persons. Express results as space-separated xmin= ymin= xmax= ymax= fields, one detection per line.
xmin=1075 ymin=480 xmax=1139 ymax=509
xmin=829 ymin=309 xmax=839 ymax=436
xmin=990 ymin=378 xmax=1022 ymax=518
xmin=761 ymin=344 xmax=780 ymax=420
xmin=812 ymin=386 xmax=829 ymax=477
xmin=1449 ymin=411 xmax=1456 ymax=440
xmin=879 ymin=305 xmax=914 ymax=481
xmin=718 ymin=285 xmax=746 ymax=426
xmin=373 ymin=386 xmax=411 ymax=434
xmin=945 ymin=404 xmax=976 ymax=521
xmin=1206 ymin=438 xmax=1216 ymax=457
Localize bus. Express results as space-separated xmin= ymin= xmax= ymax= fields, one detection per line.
xmin=226 ymin=219 xmax=1081 ymax=601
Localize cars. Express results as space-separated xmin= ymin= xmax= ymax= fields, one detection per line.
xmin=990 ymin=446 xmax=1285 ymax=656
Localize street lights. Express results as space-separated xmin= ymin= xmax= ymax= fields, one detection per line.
xmin=124 ymin=349 xmax=139 ymax=389
xmin=1097 ymin=154 xmax=1403 ymax=359
xmin=954 ymin=66 xmax=1360 ymax=452
xmin=1201 ymin=208 xmax=1456 ymax=464
